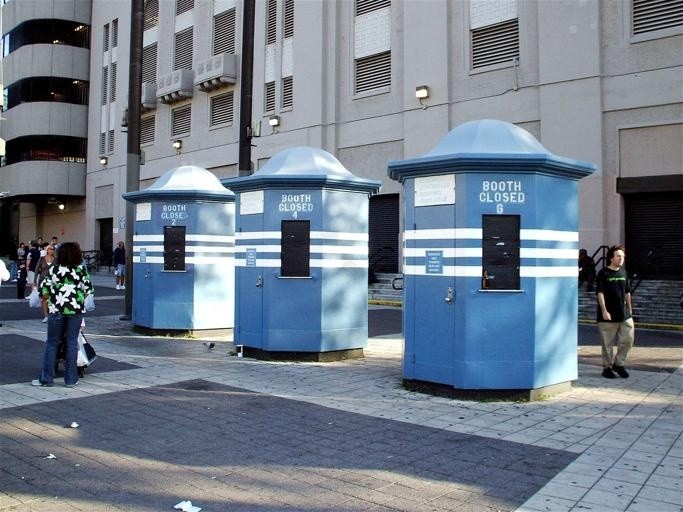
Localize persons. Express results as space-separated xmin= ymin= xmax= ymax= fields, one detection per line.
xmin=113 ymin=241 xmax=126 ymax=290
xmin=578 ymin=249 xmax=595 ymax=291
xmin=595 ymin=246 xmax=635 ymax=379
xmin=16 ymin=236 xmax=93 ymax=388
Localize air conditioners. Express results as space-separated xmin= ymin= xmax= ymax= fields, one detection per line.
xmin=141 ymin=83 xmax=156 ymax=104
xmin=156 ymin=69 xmax=193 ymax=98
xmin=194 ymin=52 xmax=237 ymax=86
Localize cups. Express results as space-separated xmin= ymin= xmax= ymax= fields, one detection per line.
xmin=235 ymin=344 xmax=244 ymax=358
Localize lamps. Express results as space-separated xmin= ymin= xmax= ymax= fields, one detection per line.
xmin=100 ymin=157 xmax=107 ymax=167
xmin=416 ymin=86 xmax=430 ymax=109
xmin=173 ymin=140 xmax=182 ymax=155
xmin=269 ymin=115 xmax=280 ymax=134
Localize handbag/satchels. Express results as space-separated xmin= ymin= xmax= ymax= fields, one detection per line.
xmin=77 ymin=330 xmax=96 ymax=367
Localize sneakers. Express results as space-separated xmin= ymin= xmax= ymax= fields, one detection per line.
xmin=116 ymin=284 xmax=125 ymax=290
xmin=32 ymin=379 xmax=51 ymax=386
xmin=612 ymin=364 xmax=629 ymax=379
xmin=602 ymin=368 xmax=616 ymax=379
